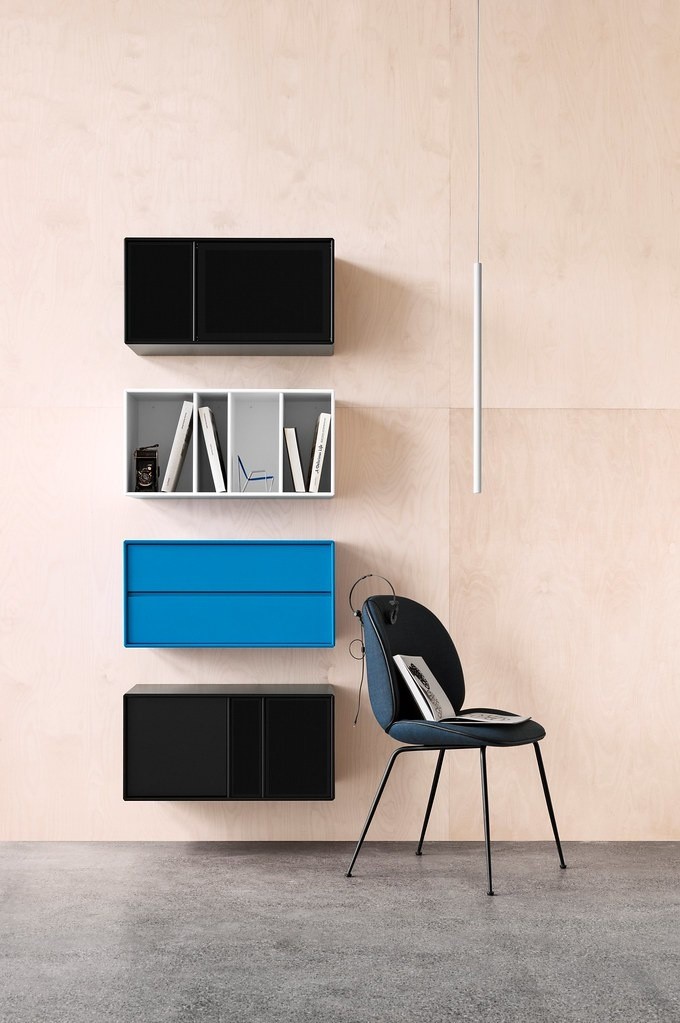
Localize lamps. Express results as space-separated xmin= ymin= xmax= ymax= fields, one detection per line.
xmin=474 ymin=0 xmax=481 ymax=494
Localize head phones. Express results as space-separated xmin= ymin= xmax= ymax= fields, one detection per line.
xmin=349 ymin=574 xmax=397 ymax=626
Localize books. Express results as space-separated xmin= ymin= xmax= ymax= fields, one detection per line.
xmin=392 ymin=654 xmax=532 ymax=725
xmin=307 ymin=412 xmax=331 ymax=492
xmin=160 ymin=401 xmax=193 ymax=492
xmin=198 ymin=406 xmax=227 ymax=492
xmin=283 ymin=427 xmax=306 ymax=492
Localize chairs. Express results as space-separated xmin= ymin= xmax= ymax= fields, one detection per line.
xmin=344 ymin=593 xmax=569 ymax=898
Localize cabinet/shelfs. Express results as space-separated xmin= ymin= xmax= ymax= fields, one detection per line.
xmin=123 ymin=684 xmax=336 ymax=802
xmin=123 ymin=234 xmax=335 ymax=358
xmin=123 ymin=540 xmax=337 ymax=650
xmin=124 ymin=389 xmax=336 ymax=498
